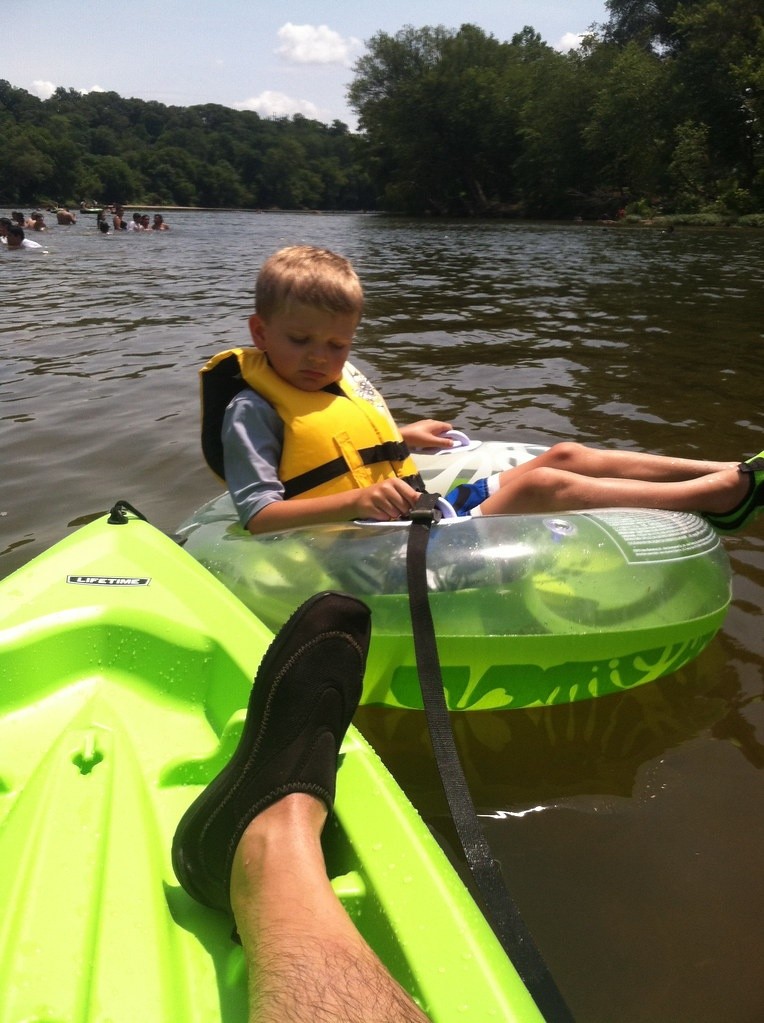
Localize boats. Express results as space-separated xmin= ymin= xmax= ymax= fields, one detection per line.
xmin=79 ymin=206 xmax=103 ymax=214
xmin=49 ymin=204 xmax=66 ymax=214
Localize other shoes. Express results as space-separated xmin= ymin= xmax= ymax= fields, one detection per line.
xmin=701 ymin=450 xmax=764 ymax=535
xmin=171 ymin=589 xmax=372 ymax=916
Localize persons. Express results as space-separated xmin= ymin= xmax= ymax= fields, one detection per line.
xmin=171 ymin=590 xmax=432 ymax=1023
xmin=219 ymin=245 xmax=764 ymax=534
xmin=0 ymin=202 xmax=171 ymax=250
